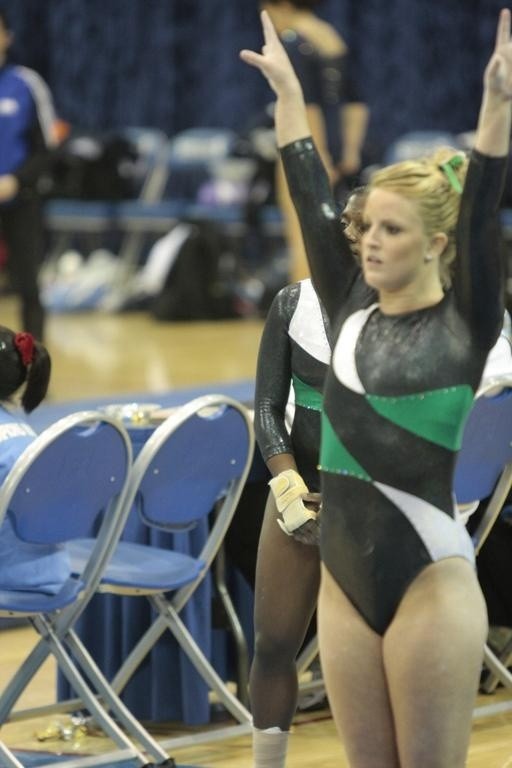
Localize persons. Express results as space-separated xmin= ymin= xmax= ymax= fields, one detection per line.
xmin=243 ymin=184 xmax=373 ymax=768
xmin=257 ymin=1 xmax=374 ymax=284
xmin=235 ymin=5 xmax=512 ymax=768
xmin=0 ymin=6 xmax=64 ymax=346
xmin=0 ymin=324 xmax=52 ymax=495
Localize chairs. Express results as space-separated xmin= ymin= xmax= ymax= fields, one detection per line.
xmin=0 ymin=412 xmax=152 ymax=768
xmin=297 ymin=380 xmax=512 ymax=695
xmin=0 ymin=394 xmax=254 ymax=768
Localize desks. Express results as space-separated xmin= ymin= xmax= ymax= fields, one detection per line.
xmin=20 ymin=382 xmax=254 ymax=726
